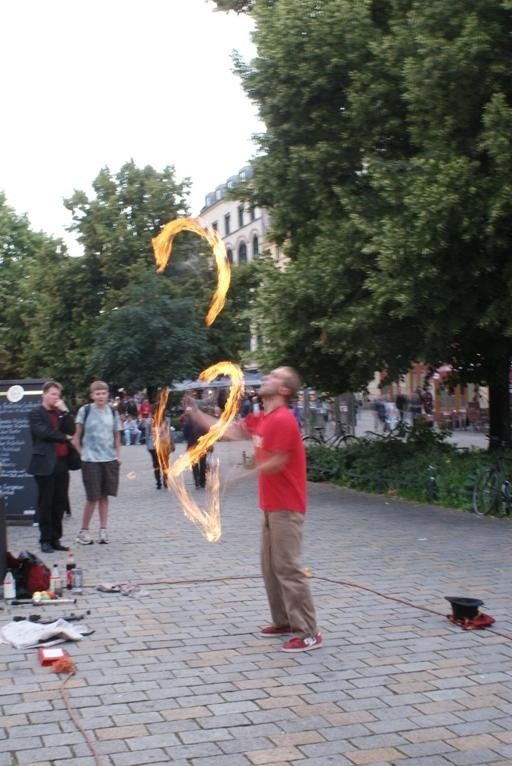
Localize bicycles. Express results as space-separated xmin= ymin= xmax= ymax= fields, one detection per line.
xmin=364 ymin=419 xmax=418 ymax=442
xmin=472 ymin=434 xmax=512 ymax=516
xmin=302 ymin=421 xmax=362 ymax=448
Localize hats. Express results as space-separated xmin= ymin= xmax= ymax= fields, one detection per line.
xmin=443 ymin=595 xmax=483 ymax=620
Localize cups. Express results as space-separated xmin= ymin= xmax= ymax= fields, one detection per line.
xmin=71 ymin=568 xmax=84 ymax=593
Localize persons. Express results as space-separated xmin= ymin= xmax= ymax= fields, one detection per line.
xmin=396 ymin=387 xmax=408 ymax=420
xmin=374 ymin=388 xmax=392 ymax=434
xmin=422 ymin=384 xmax=433 ymax=416
xmin=112 ymin=385 xmax=358 ymax=488
xmin=28 ymin=382 xmax=76 ymax=554
xmin=180 ymin=364 xmax=325 ymax=653
xmin=71 ymin=381 xmax=124 ymax=545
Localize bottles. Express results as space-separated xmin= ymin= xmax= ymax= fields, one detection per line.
xmin=3 ymin=568 xmax=17 ymax=600
xmin=65 ymin=552 xmax=77 ymax=590
xmin=49 ymin=564 xmax=62 ymax=591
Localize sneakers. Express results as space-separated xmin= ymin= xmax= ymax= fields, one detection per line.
xmin=281 ymin=633 xmax=324 ymax=654
xmin=39 ymin=530 xmax=110 ymax=554
xmin=258 ymin=625 xmax=292 ymax=638
